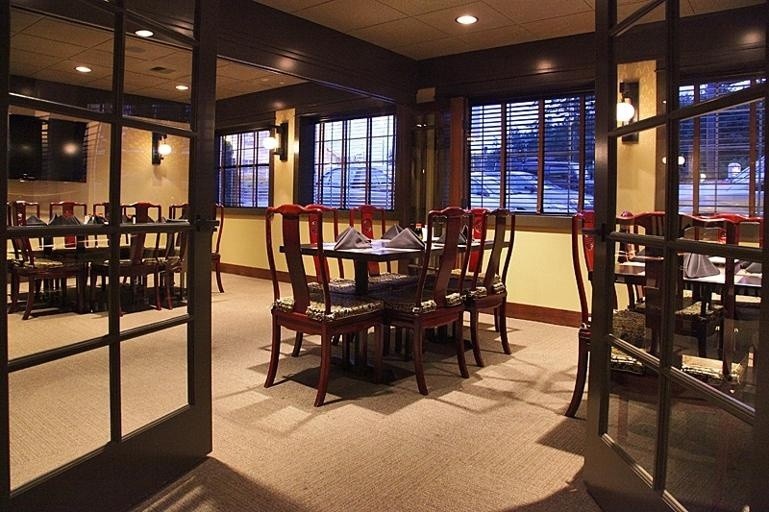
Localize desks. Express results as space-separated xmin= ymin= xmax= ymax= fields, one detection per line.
xmin=280 ymin=235 xmax=509 ymax=364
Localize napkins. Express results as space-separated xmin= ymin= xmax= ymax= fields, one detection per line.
xmin=333 ymin=228 xmax=371 ymax=251
xmin=379 ymin=223 xmax=425 ymax=248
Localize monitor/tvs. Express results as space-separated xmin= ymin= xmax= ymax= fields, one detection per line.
xmin=8 ymin=112 xmax=89 ymax=183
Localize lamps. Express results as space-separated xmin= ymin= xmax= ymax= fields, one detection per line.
xmin=615 ymin=81 xmax=639 ymax=143
xmin=262 ymin=119 xmax=288 ymax=163
xmin=151 ymin=131 xmax=173 ymax=164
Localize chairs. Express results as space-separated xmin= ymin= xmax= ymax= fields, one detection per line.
xmin=441 ymin=207 xmax=515 ymax=366
xmin=349 ymin=205 xmax=417 ymax=352
xmin=265 ymin=202 xmax=385 ymax=407
xmin=7 ymin=199 xmax=224 ymax=320
xmin=565 ymin=207 xmax=764 ymax=421
xmin=290 ymin=202 xmax=382 ymax=369
xmin=373 ymin=208 xmax=473 ymax=395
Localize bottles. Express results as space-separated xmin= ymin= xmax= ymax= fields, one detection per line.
xmin=415 ymin=223 xmax=423 ymax=240
